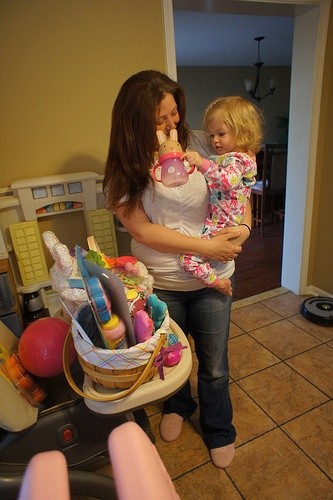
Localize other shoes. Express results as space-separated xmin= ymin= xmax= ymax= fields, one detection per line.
xmin=210 ymin=442 xmax=235 ymax=467
xmin=159 ymin=413 xmax=184 ymax=441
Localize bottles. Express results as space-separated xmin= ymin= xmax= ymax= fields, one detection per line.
xmin=151 ymin=128 xmax=195 ymax=187
xmin=101 ymin=313 xmax=128 ymax=350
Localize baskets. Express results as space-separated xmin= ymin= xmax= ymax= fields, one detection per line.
xmin=63 ymin=300 xmax=169 ymax=401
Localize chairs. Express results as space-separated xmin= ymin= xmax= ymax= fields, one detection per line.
xmin=251 ymin=144 xmax=287 ymax=240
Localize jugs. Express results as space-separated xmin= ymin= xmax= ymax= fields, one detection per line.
xmin=22 ymin=291 xmax=43 ymax=321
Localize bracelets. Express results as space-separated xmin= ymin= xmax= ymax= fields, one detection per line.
xmin=241 ymin=223 xmax=252 ymax=235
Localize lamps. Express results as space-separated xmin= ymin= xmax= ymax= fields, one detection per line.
xmin=244 ymin=36 xmax=278 ymax=103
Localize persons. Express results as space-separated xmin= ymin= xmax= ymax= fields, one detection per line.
xmin=178 ymin=96 xmax=263 ymax=297
xmin=103 ymin=68 xmax=251 ymax=468
